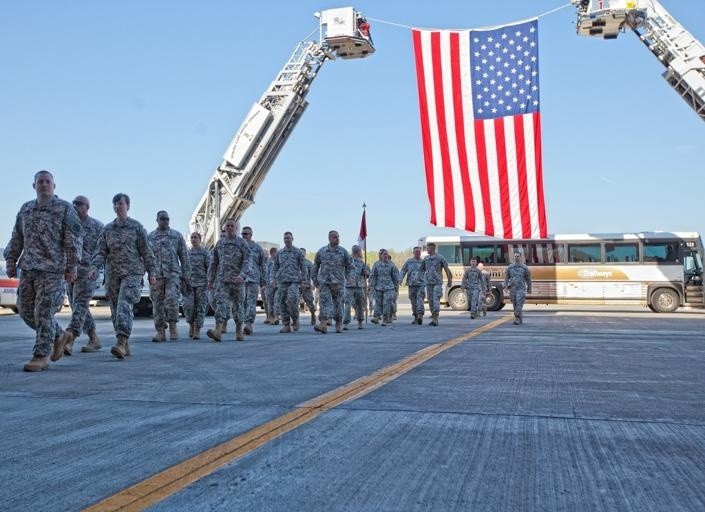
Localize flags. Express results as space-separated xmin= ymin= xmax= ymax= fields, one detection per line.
xmin=410 ymin=15 xmax=548 ymax=241
xmin=357 ymin=210 xmax=367 ymax=251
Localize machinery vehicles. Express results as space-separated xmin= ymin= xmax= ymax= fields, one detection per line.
xmin=90 ymin=5 xmax=376 ymax=317
xmin=574 ymin=0 xmax=705 ymax=122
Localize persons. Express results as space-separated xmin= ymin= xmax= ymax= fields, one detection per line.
xmin=461 ymin=253 xmax=495 ymax=319
xmin=63 ymin=194 xmax=356 ymax=360
xmin=506 ymin=251 xmax=534 ymax=326
xmin=4 ymin=168 xmax=83 ymax=372
xmin=665 ymin=245 xmax=674 ymax=262
xmin=342 ymin=243 xmax=453 ymax=330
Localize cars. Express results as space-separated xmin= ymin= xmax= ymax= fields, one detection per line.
xmin=0 ymin=247 xmax=65 ymax=316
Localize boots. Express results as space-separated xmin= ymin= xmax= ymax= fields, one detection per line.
xmin=513 ymin=316 xmax=523 ymax=324
xmin=471 ymin=308 xmax=487 ymax=319
xmin=311 ymin=313 xmax=438 ymax=332
xmin=24 ymin=318 xmax=300 ymax=371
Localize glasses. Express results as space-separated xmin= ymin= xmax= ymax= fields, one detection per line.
xmin=160 ymin=217 xmax=169 ymax=221
xmin=72 ymin=201 xmax=83 ymax=206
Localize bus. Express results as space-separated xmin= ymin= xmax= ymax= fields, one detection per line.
xmin=415 ymin=229 xmax=705 ymax=313
